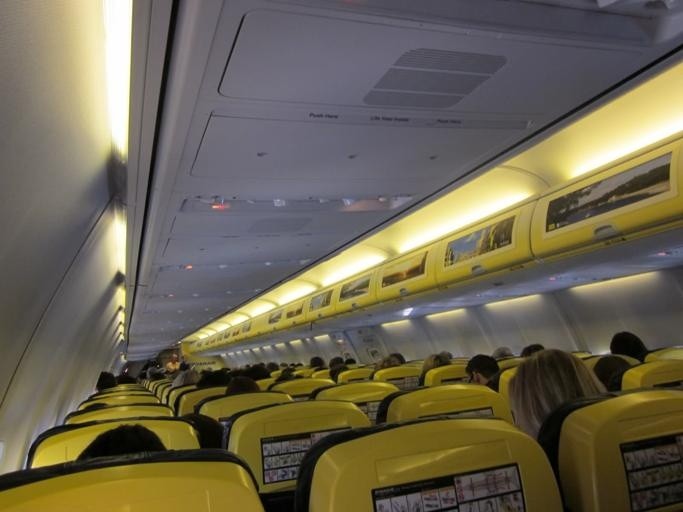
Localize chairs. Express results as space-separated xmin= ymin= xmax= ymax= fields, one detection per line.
xmin=0 ymin=345 xmax=683 ymax=512
